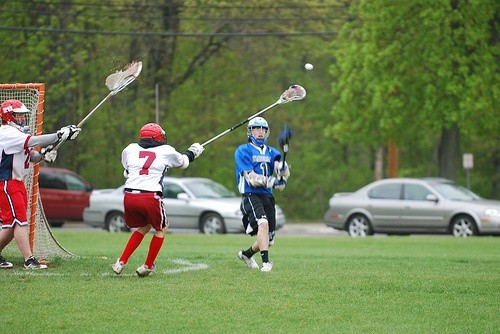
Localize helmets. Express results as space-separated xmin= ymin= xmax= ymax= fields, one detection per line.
xmin=246 ymin=117 xmax=270 ymax=146
xmin=0 ymin=99 xmax=30 ymax=133
xmin=139 ymin=123 xmax=167 ymax=144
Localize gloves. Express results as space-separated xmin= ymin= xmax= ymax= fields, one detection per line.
xmin=187 ymin=142 xmax=205 ymax=160
xmin=277 ymin=161 xmax=290 ymax=181
xmin=56 ymin=124 xmax=82 ymax=141
xmin=266 ymin=176 xmax=286 ymax=191
xmin=40 ymin=145 xmax=58 ymax=163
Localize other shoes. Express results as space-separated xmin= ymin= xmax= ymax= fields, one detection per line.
xmin=136 ymin=262 xmax=156 ymax=277
xmin=111 ymin=257 xmax=127 ymax=274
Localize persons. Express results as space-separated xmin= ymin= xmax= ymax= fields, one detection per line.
xmin=235 ymin=117 xmax=290 ymax=272
xmin=0 ymin=99 xmax=81 ymax=270
xmin=109 ymin=124 xmax=205 ymax=277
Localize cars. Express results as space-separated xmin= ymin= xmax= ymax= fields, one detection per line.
xmin=325 ymin=178 xmax=500 ymax=238
xmin=82 ymin=177 xmax=286 ymax=236
xmin=36 ymin=166 xmax=94 ymax=227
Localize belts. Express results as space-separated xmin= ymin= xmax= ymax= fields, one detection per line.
xmin=125 ymin=188 xmax=150 ymax=193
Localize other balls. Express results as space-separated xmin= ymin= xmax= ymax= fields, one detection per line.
xmin=305 ymin=63 xmax=313 ymax=71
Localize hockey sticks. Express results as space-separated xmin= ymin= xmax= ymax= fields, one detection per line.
xmin=52 ymin=60 xmax=144 ymax=150
xmin=201 ymin=84 xmax=306 ymax=148
xmin=277 ymin=123 xmax=292 ymax=181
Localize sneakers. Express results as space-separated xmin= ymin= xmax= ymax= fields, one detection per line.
xmin=22 ymin=255 xmax=48 ymax=270
xmin=0 ymin=256 xmax=13 ymax=269
xmin=261 ymin=262 xmax=272 ymax=272
xmin=238 ymin=250 xmax=259 ymax=270
